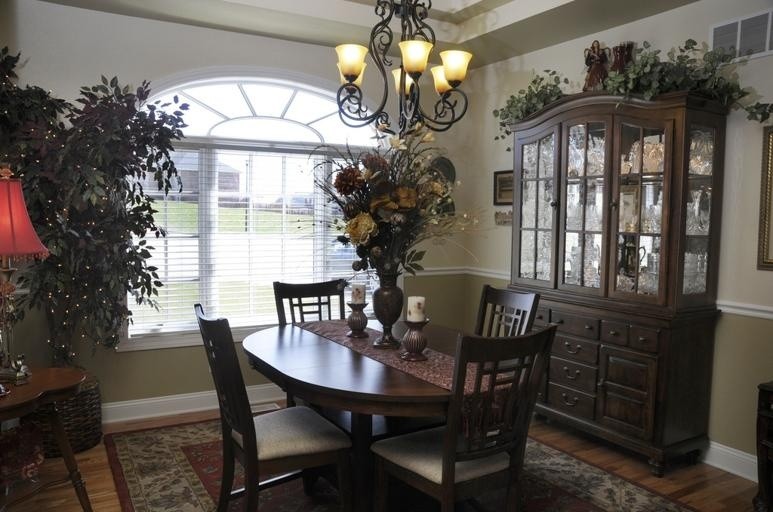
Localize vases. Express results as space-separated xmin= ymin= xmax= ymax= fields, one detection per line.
xmin=294 ymin=121 xmax=481 ymax=273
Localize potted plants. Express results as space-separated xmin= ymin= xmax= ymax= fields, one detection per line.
xmin=758 ymin=126 xmax=773 ymax=270
xmin=494 ymin=170 xmax=515 ymax=207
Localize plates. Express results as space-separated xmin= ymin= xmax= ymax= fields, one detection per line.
xmin=504 ymin=91 xmax=730 ymax=479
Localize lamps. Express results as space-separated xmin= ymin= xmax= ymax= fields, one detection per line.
xmin=369 ymin=321 xmax=562 ymax=512
xmin=474 ymin=285 xmax=541 ymax=337
xmin=192 ymin=300 xmax=352 ymax=512
xmin=272 ymin=279 xmax=345 ymax=325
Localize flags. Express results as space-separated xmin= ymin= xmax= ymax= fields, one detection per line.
xmin=373 ymin=273 xmax=403 ymax=349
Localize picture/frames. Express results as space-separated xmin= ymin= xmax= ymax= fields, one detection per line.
xmin=0 ymin=366 xmax=93 ymax=512
xmin=752 ymin=380 xmax=773 ymax=512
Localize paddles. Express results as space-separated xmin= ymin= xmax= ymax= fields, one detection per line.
xmin=105 ymin=407 xmax=699 ymax=512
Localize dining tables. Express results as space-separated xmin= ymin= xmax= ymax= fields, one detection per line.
xmin=0 ymin=42 xmax=190 ymax=456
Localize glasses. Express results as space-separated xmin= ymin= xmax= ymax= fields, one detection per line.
xmin=0 ymin=163 xmax=50 ymax=386
xmin=335 ymin=0 xmax=472 ymax=135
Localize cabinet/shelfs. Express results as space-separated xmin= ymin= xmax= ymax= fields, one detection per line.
xmin=521 ymin=134 xmax=714 ymax=299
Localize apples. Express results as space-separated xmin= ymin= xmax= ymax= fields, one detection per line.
xmin=407 ymin=296 xmax=426 ymax=323
xmin=351 ymin=282 xmax=366 ymax=304
